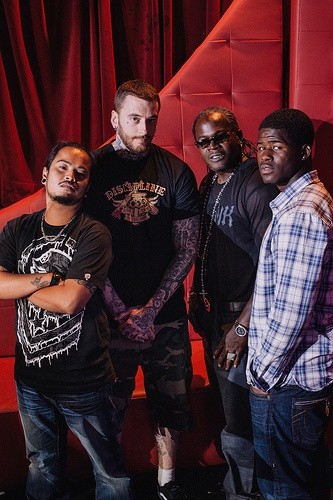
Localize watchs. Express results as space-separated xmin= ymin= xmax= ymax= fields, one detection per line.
xmin=235 ymin=319 xmax=248 ymax=337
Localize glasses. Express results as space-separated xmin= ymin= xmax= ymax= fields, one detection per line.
xmin=194 ymin=131 xmax=236 ymax=149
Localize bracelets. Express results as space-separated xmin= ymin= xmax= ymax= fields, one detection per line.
xmin=112 ymin=313 xmax=128 ymax=320
xmin=50 ymin=271 xmax=60 ymax=286
xmin=189 ymin=286 xmax=199 ymax=298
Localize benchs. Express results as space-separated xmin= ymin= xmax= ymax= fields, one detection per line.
xmin=0 ymin=339 xmax=333 ymax=490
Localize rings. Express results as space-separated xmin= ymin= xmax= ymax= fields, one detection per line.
xmin=227 ymin=353 xmax=237 ymax=361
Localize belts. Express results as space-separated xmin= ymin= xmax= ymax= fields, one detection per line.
xmin=202 ymin=297 xmax=246 ymax=312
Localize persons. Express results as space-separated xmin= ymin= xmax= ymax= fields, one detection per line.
xmin=0 ymin=142 xmax=133 ymax=500
xmin=187 ymin=106 xmax=279 ymax=500
xmin=89 ymin=80 xmax=199 ymax=500
xmin=248 ymin=109 xmax=332 ymax=499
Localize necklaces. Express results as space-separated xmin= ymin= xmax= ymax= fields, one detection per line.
xmin=197 ymin=152 xmax=246 ymax=312
xmin=41 ymin=210 xmax=79 ymax=241
